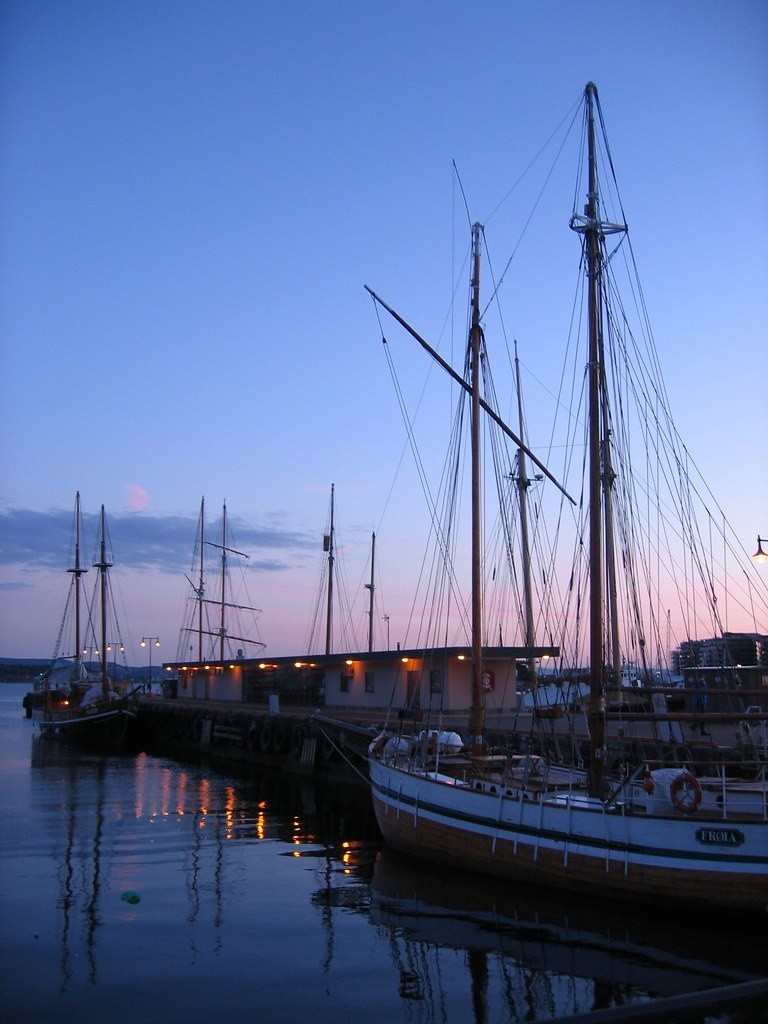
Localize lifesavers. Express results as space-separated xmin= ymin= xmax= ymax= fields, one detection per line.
xmin=234 ymin=719 xmax=248 ymax=748
xmin=670 ymin=773 xmax=702 ymax=814
xmin=260 ymin=725 xmax=274 ymax=752
xmin=246 ymin=722 xmax=262 ymax=752
xmin=321 ymin=735 xmax=340 ymax=762
xmin=289 ymin=725 xmax=308 ymax=755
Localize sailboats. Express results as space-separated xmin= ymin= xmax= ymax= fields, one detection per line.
xmin=164 ymin=80 xmax=767 ymax=927
xmin=21 ymin=488 xmax=148 ymax=743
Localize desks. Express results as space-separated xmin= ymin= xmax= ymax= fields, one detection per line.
xmin=471 ymin=754 xmax=545 ymax=776
